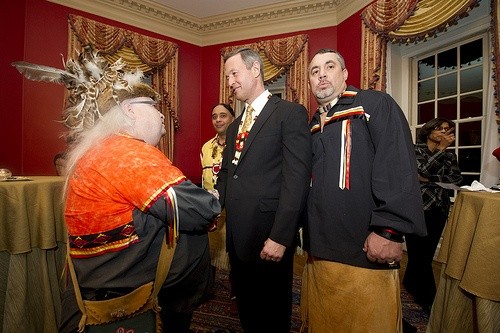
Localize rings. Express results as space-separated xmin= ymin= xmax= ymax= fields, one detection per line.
xmin=388 ymin=260 xmax=395 ymax=265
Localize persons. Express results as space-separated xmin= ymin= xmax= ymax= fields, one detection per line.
xmin=54 ymin=152 xmax=69 ymax=176
xmin=402 ymin=117 xmax=464 ymax=316
xmin=208 ymin=47 xmax=313 ymax=333
xmin=296 ymin=48 xmax=428 ymax=333
xmin=199 ymin=102 xmax=238 ymax=317
xmin=62 ymin=80 xmax=222 ymax=333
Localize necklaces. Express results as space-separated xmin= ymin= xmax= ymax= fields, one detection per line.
xmin=217 ymin=137 xmax=227 ymax=146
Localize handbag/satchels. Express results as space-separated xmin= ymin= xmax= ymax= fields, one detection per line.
xmin=76 ymin=281 xmax=163 ymax=333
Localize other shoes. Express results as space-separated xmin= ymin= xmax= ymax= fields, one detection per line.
xmin=229 ymin=301 xmax=238 ymax=315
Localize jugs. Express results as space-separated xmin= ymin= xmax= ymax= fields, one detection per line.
xmin=0 ymin=169 xmax=12 ymax=182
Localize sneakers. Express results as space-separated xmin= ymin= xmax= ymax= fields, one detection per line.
xmin=214 ymin=280 xmax=232 ymax=299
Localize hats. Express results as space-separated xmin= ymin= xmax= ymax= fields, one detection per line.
xmin=65 ymin=72 xmax=161 ymax=131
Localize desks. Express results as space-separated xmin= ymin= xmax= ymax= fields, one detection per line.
xmin=0 ymin=176 xmax=80 ymax=333
xmin=425 ymin=188 xmax=500 ymax=333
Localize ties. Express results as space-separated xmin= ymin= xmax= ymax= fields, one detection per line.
xmin=240 ymin=106 xmax=255 ymax=133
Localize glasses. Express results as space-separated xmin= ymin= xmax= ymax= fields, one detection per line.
xmin=129 ymin=99 xmax=161 ymax=111
xmin=435 ymin=126 xmax=449 ymax=131
